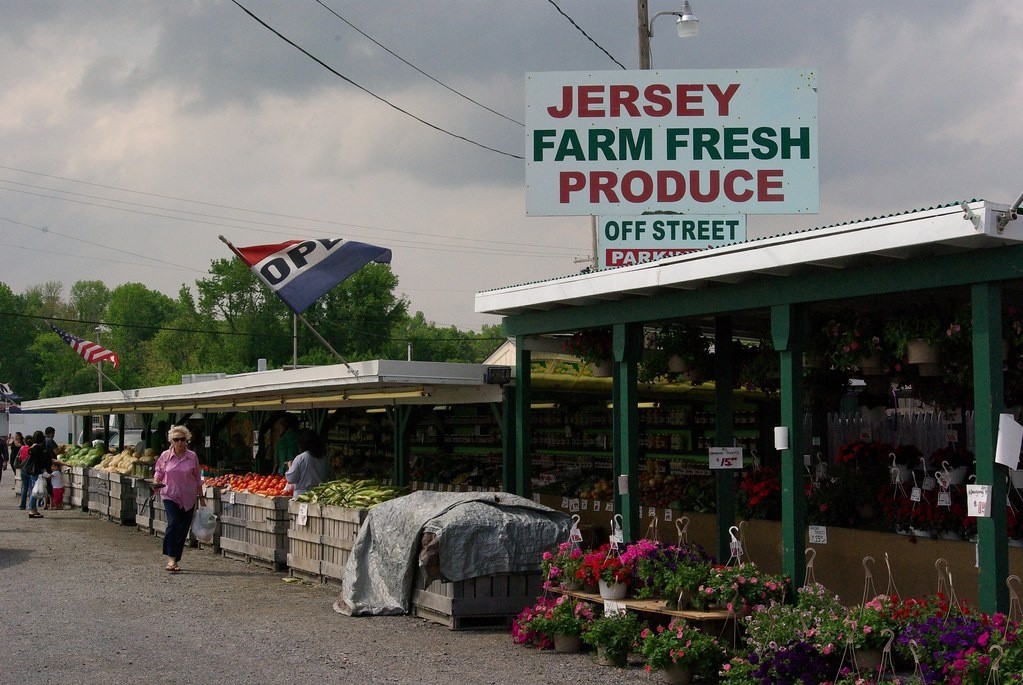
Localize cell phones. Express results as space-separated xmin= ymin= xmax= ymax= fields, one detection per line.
xmin=9 ymin=433 xmax=11 ymax=436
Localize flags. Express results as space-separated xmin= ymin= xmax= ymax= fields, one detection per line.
xmin=49 ymin=323 xmax=119 ymax=368
xmin=227 ymin=239 xmax=392 ymax=315
xmin=0 ymin=383 xmax=23 ymax=399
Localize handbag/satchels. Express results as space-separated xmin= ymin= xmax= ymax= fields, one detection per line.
xmin=191 ymin=504 xmax=217 ymax=541
xmin=13 ymin=456 xmax=22 ymax=469
xmin=19 ymin=455 xmax=36 ymax=475
xmin=31 ymin=472 xmax=51 ymax=499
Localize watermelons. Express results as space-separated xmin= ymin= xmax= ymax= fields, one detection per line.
xmin=58 ymin=442 xmax=104 ymax=467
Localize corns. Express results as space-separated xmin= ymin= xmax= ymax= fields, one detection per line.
xmin=297 ymin=478 xmax=412 ymax=510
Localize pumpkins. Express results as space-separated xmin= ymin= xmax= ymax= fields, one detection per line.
xmin=93 ymin=448 xmax=161 ymax=474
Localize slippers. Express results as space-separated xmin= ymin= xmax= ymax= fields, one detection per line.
xmin=165 ymin=564 xmax=181 ymax=570
xmin=29 ymin=512 xmax=44 ymax=518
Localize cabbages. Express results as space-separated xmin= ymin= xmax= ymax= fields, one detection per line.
xmin=667 ymin=476 xmax=715 ymax=517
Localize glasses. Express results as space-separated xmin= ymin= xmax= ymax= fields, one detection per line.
xmin=172 ymin=437 xmax=186 ymax=442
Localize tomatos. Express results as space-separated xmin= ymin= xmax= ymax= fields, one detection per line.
xmin=232 ymin=472 xmax=294 ymax=497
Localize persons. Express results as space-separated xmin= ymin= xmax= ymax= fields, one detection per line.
xmin=258 ymin=410 xmax=389 ymax=499
xmin=150 ymin=425 xmax=207 ymax=571
xmin=0 ymin=427 xmax=68 ymax=518
xmin=135 ymin=413 xmax=252 ymax=469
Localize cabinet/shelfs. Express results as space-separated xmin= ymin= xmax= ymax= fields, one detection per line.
xmin=377 ymin=421 xmax=504 ymax=449
xmin=534 ymin=399 xmax=763 ymax=455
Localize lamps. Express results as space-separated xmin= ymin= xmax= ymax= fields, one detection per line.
xmin=530 ymin=403 xmax=560 ymax=409
xmin=606 ymin=402 xmax=661 ymax=409
xmin=481 ymin=365 xmax=511 ymax=388
xmin=364 ymin=406 xmax=397 ymax=414
xmin=56 ymin=384 xmax=434 ymax=415
xmin=431 ymin=406 xmax=452 ymax=411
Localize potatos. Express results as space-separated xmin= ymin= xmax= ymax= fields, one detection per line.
xmin=205 ymin=473 xmax=239 ymax=487
xmin=639 ymin=482 xmax=684 ymax=510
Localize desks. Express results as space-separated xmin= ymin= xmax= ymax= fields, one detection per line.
xmin=536 ymin=584 xmax=748 ymax=636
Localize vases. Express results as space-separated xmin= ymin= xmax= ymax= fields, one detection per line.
xmin=893 ymin=524 xmax=938 ymax=537
xmin=918 ymin=363 xmax=942 ymax=377
xmin=861 ymin=359 xmax=888 ymax=375
xmin=802 ymin=354 xmax=823 ymax=368
xmin=1003 ymin=340 xmax=1010 ymax=360
xmin=668 ymin=354 xmax=693 ymax=372
xmin=905 ymin=338 xmax=942 ymax=363
xmin=935 ymin=530 xmax=963 ymax=540
xmin=662 ymin=662 xmax=696 ymax=685
xmin=597 ymin=645 xmax=628 ymax=666
xmin=1007 ymin=537 xmax=1023 ymax=547
xmin=592 ymin=360 xmax=612 ymax=377
xmin=553 ymin=633 xmax=582 ymax=653
xmin=853 ymin=647 xmax=895 ymax=670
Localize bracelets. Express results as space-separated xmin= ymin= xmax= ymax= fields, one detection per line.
xmin=199 ymin=496 xmax=204 ymax=498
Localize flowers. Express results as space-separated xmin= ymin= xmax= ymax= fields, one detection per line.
xmin=816 ymin=296 xmax=1023 ymax=385
xmin=510 ymin=433 xmax=1023 ymax=685
